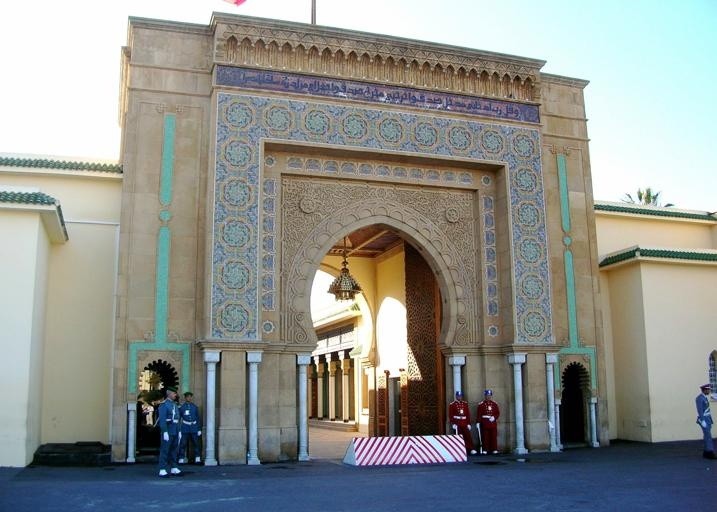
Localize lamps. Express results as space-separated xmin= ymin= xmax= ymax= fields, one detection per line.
xmin=326 ymin=235 xmax=365 ymax=302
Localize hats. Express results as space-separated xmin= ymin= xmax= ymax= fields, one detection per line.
xmin=456 ymin=391 xmax=462 ymax=396
xmin=484 ymin=390 xmax=492 ymax=395
xmin=167 ymin=385 xmax=178 ymax=392
xmin=183 ymin=392 xmax=193 ymax=396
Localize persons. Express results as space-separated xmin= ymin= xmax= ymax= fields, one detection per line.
xmin=447 ymin=391 xmax=478 ymax=455
xmin=475 ymin=389 xmax=500 ymax=455
xmin=695 ymin=383 xmax=717 ymax=460
xmin=177 ymin=392 xmax=203 ymax=464
xmin=157 ymin=386 xmax=182 ymax=477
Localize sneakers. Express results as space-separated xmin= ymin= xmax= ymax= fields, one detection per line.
xmin=470 ymin=449 xmax=499 ymax=455
xmin=195 ymin=457 xmax=201 ymax=463
xmin=159 ymin=457 xmax=184 ymax=478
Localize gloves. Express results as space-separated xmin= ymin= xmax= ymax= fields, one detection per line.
xmin=489 ymin=416 xmax=495 ymax=423
xmin=178 ymin=431 xmax=182 ymax=439
xmin=452 ymin=423 xmax=480 ymax=431
xmin=198 ymin=430 xmax=201 ymax=436
xmin=163 ymin=432 xmax=169 ymax=442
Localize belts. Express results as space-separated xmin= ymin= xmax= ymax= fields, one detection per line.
xmin=453 ymin=416 xmax=467 ymax=419
xmin=483 ymin=415 xmax=491 ymax=418
xmin=182 ymin=420 xmax=196 ymax=425
xmin=167 ymin=419 xmax=180 ymax=423
